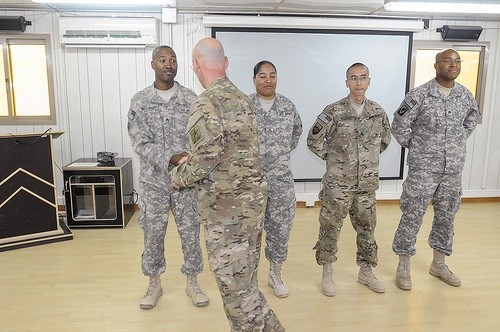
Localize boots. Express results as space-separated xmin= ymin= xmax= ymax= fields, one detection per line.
xmin=140 ymin=275 xmax=163 ymax=309
xmin=268 ymin=262 xmax=288 ymax=298
xmin=358 ymin=266 xmax=385 ymax=293
xmin=430 ymin=249 xmax=461 ymax=286
xmin=396 ymin=255 xmax=412 ymax=290
xmin=322 ymin=263 xmax=335 ymax=296
xmin=186 ymin=275 xmax=209 ymax=307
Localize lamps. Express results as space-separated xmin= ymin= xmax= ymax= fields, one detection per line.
xmin=383 ymin=0 xmax=500 ymax=15
xmin=437 ymin=24 xmax=484 ymax=43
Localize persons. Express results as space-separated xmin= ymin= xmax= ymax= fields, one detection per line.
xmin=127 ymin=45 xmax=209 ymax=309
xmin=306 ymin=63 xmax=391 ymax=297
xmin=391 ymin=48 xmax=482 ymax=290
xmin=244 ymin=60 xmax=303 ymax=298
xmin=170 ymin=37 xmax=286 ymax=332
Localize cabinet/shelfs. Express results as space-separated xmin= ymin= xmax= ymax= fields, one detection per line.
xmin=63 ymin=157 xmax=136 ymax=229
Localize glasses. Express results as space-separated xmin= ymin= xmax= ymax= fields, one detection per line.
xmin=435 ymin=59 xmax=463 ymax=64
xmin=348 ymin=76 xmax=369 ymax=81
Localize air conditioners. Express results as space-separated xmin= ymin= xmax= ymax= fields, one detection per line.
xmin=57 ymin=15 xmax=160 ymax=50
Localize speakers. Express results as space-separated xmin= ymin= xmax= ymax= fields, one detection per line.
xmin=441 ymin=25 xmax=483 ymax=40
xmin=0 ymin=16 xmax=26 ymax=32
